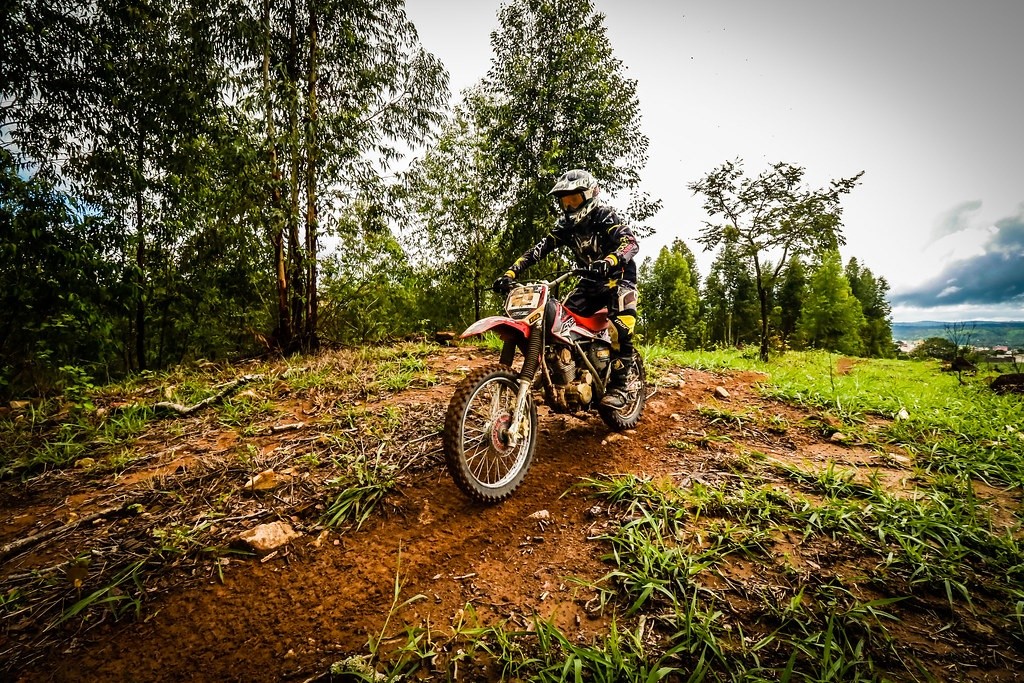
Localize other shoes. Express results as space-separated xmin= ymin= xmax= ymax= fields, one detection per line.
xmin=602 ymin=388 xmax=632 ymax=409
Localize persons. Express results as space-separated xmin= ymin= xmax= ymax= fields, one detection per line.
xmin=493 ymin=169 xmax=640 ymax=409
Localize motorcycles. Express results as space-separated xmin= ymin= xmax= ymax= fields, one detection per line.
xmin=443 ymin=264 xmax=647 ymax=505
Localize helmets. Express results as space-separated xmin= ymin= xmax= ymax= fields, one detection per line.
xmin=545 ymin=170 xmax=600 ymax=227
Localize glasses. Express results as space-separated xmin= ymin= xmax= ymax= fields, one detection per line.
xmin=557 ymin=192 xmax=586 ymax=213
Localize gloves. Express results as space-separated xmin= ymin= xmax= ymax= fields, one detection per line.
xmin=495 ymin=272 xmax=515 ymax=287
xmin=590 ymin=260 xmax=614 ymax=275
